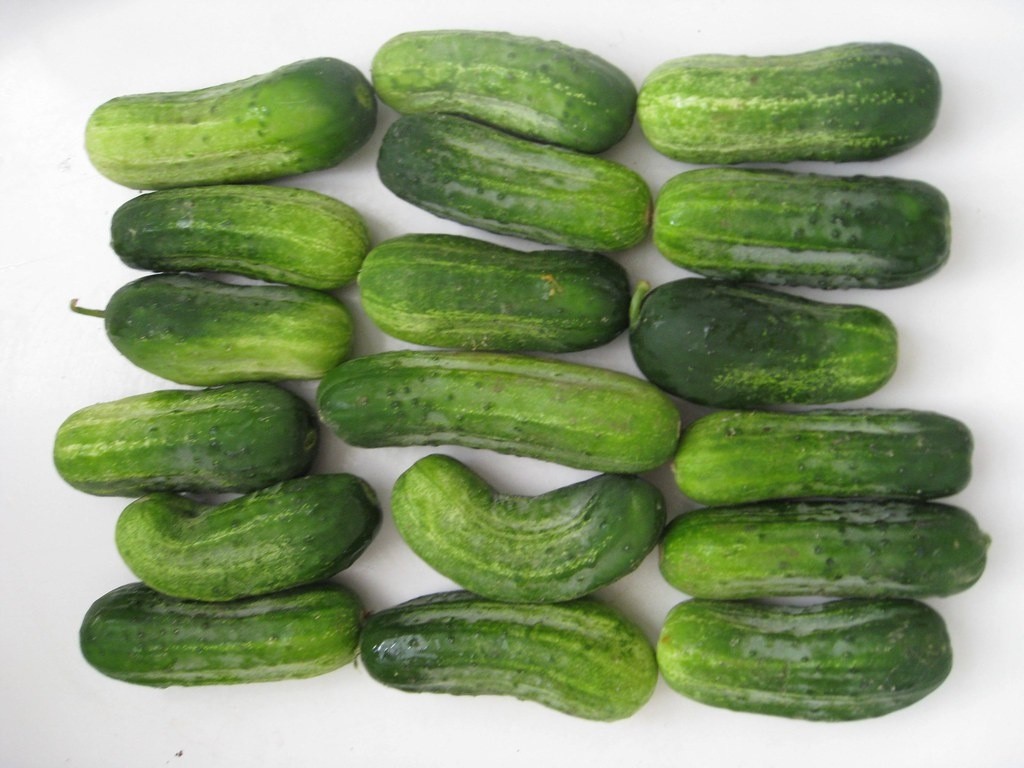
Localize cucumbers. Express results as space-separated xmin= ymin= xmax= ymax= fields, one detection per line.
xmin=52 ymin=28 xmax=994 ymax=722
